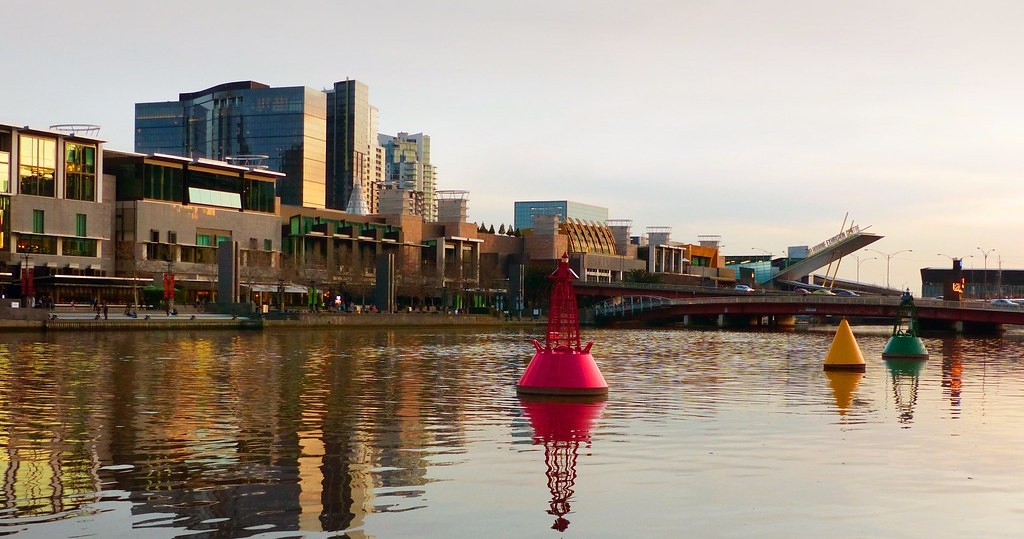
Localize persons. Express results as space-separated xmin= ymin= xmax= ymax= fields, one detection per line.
xmin=103 ymin=302 xmax=108 ymax=320
xmin=146 ymin=298 xmax=150 ymax=309
xmin=93 ymin=296 xmax=98 ymax=310
xmin=31 ymin=296 xmax=35 ymax=309
xmin=140 ymin=298 xmax=144 ymax=310
xmin=41 ymin=295 xmax=46 ymax=307
xmin=47 ymin=296 xmax=52 ymax=310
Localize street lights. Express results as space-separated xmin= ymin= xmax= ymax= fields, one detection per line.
xmin=702 ymin=245 xmax=726 ymax=297
xmin=18 ymin=244 xmax=39 ymax=305
xmin=976 ymin=247 xmax=995 ymax=305
xmin=864 ymin=248 xmax=914 ymax=296
xmin=857 ymin=257 xmax=879 ymax=295
xmin=752 ymin=247 xmax=772 ymax=290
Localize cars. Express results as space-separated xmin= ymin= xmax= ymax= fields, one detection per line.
xmin=734 ymin=285 xmax=755 ymax=292
xmin=837 ymin=291 xmax=860 ymax=297
xmin=812 ymin=289 xmax=836 ymax=296
xmin=794 ymin=289 xmax=812 ymax=296
xmin=992 ymin=300 xmax=1019 ymax=308
xmin=931 ymin=296 xmax=944 ymax=303
